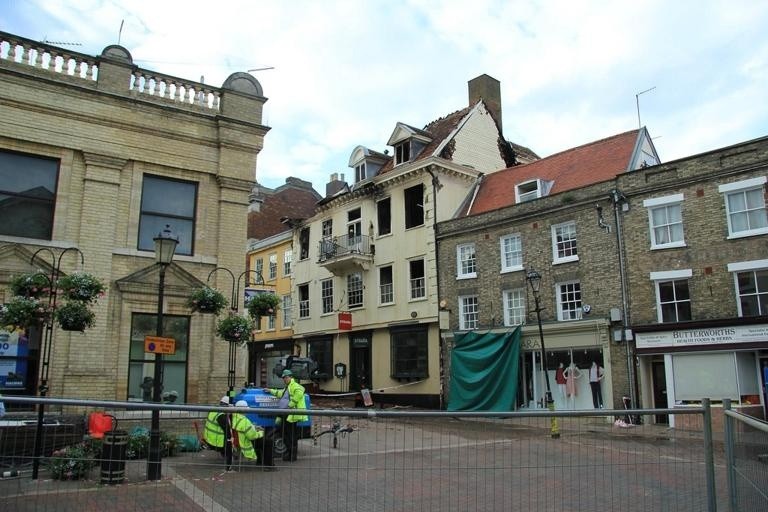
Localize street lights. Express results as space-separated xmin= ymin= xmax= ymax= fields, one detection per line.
xmin=147 ymin=223 xmax=180 ymax=481
xmin=525 ymin=266 xmax=560 ymax=439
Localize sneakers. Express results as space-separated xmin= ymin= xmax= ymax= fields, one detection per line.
xmin=264 ymin=468 xmax=278 ymax=472
xmin=281 ymin=451 xmax=296 ymax=461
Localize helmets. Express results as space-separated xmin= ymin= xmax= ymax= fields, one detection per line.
xmin=235 ymin=401 xmax=248 ymax=407
xmin=221 ymin=396 xmax=229 ymax=404
xmin=282 ymin=370 xmax=292 ymax=377
xmin=163 ymin=391 xmax=178 ymax=398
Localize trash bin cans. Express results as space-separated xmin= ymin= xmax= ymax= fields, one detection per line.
xmin=101 ymin=430 xmax=129 ymax=484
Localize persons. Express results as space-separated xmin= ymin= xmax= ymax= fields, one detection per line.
xmin=554 ymin=362 xmax=567 ymax=409
xmin=202 ymin=395 xmax=230 ymax=458
xmin=262 ymin=369 xmax=309 ymax=462
xmin=231 ymin=399 xmax=281 ymax=472
xmin=563 ymin=362 xmax=583 ymax=410
xmin=588 ymin=362 xmax=606 ymax=409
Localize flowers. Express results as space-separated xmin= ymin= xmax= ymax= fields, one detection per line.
xmin=184 ymin=285 xmax=282 ymax=344
xmin=45 ymin=440 xmax=94 ymax=478
xmin=0 ymin=271 xmax=107 ymax=335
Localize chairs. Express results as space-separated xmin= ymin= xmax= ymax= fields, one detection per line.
xmin=229 ymin=428 xmax=242 ymax=472
xmin=192 ymin=421 xmax=220 ymax=459
